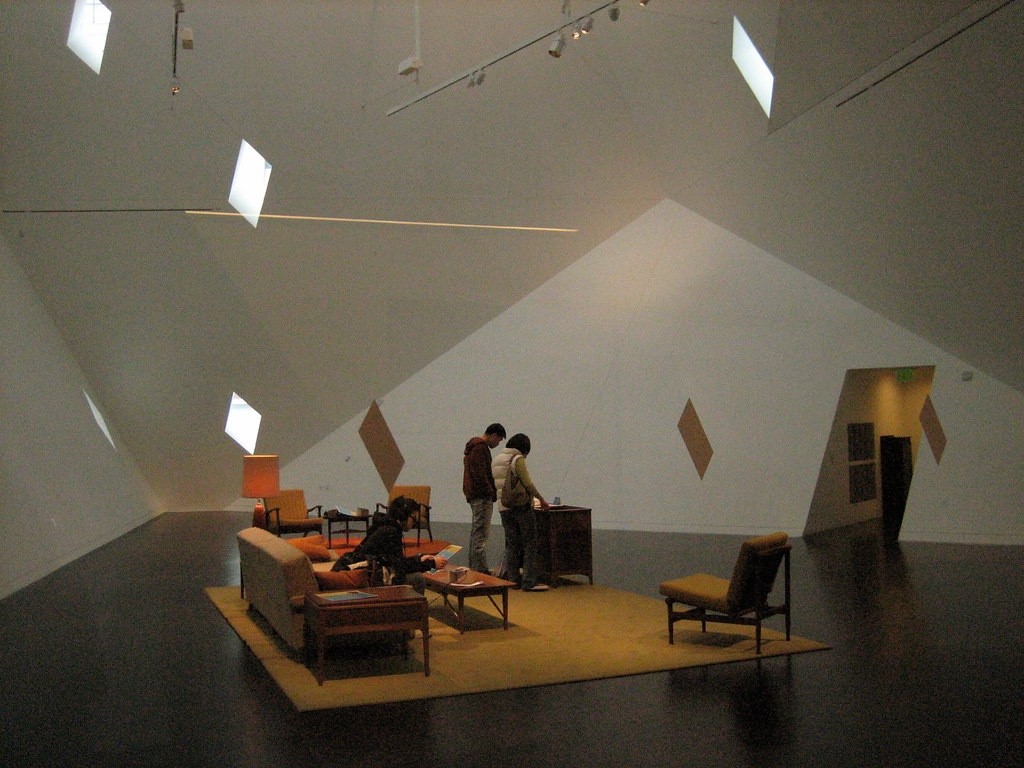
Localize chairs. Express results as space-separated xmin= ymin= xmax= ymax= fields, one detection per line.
xmin=659 ymin=531 xmax=793 ymax=654
xmin=375 ymin=484 xmax=432 ymax=548
xmin=263 ymin=489 xmax=323 ymax=537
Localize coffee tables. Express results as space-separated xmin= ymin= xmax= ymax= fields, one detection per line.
xmin=414 ymin=564 xmax=517 ymax=635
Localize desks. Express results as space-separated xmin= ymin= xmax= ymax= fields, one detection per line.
xmin=324 ymin=513 xmax=373 ymax=548
xmin=520 ymin=506 xmax=593 ymax=589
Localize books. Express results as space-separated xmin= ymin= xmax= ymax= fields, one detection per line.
xmin=426 ymin=545 xmax=462 ymax=575
xmin=533 ymin=499 xmax=563 ymax=510
xmin=315 ymin=590 xmax=379 ymax=602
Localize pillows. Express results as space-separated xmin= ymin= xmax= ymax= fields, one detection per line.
xmin=312 ymin=570 xmax=367 ymax=589
xmin=294 ymin=542 xmax=331 ymax=563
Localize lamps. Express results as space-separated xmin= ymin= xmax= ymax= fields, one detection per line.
xmin=549 ymin=27 xmax=566 ymax=58
xmin=466 ymin=69 xmax=486 ymax=88
xmin=571 ymin=21 xmax=582 ymax=39
xmin=169 ymin=74 xmax=180 ymax=92
xmin=242 ymin=454 xmax=279 ymax=528
xmin=581 ymin=13 xmax=594 ymax=34
xmin=608 ymin=2 xmax=621 ymax=21
xmin=639 ymin=0 xmax=650 ymax=6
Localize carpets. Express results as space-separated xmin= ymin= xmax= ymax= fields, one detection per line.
xmin=204 ymin=569 xmax=834 ymax=714
xmin=323 ymin=539 xmax=451 ymax=559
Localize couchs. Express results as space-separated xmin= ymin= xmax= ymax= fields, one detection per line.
xmin=237 ymin=527 xmax=415 ymax=669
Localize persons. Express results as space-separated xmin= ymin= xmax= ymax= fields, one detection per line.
xmin=491 ymin=433 xmax=551 ymax=590
xmin=356 ymin=496 xmax=447 ymax=592
xmin=462 ymin=423 xmax=507 ymax=575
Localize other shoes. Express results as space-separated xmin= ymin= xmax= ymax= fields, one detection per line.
xmin=527 ymin=583 xmax=548 ymax=590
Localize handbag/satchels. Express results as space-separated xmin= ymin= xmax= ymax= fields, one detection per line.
xmin=500 ymin=454 xmax=531 ymax=508
xmin=331 ymin=553 xmax=383 ymax=587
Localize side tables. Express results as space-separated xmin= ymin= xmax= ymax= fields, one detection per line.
xmin=303 ymin=586 xmax=429 ymax=686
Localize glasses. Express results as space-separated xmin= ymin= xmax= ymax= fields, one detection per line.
xmin=409 ymin=514 xmax=417 ymax=522
